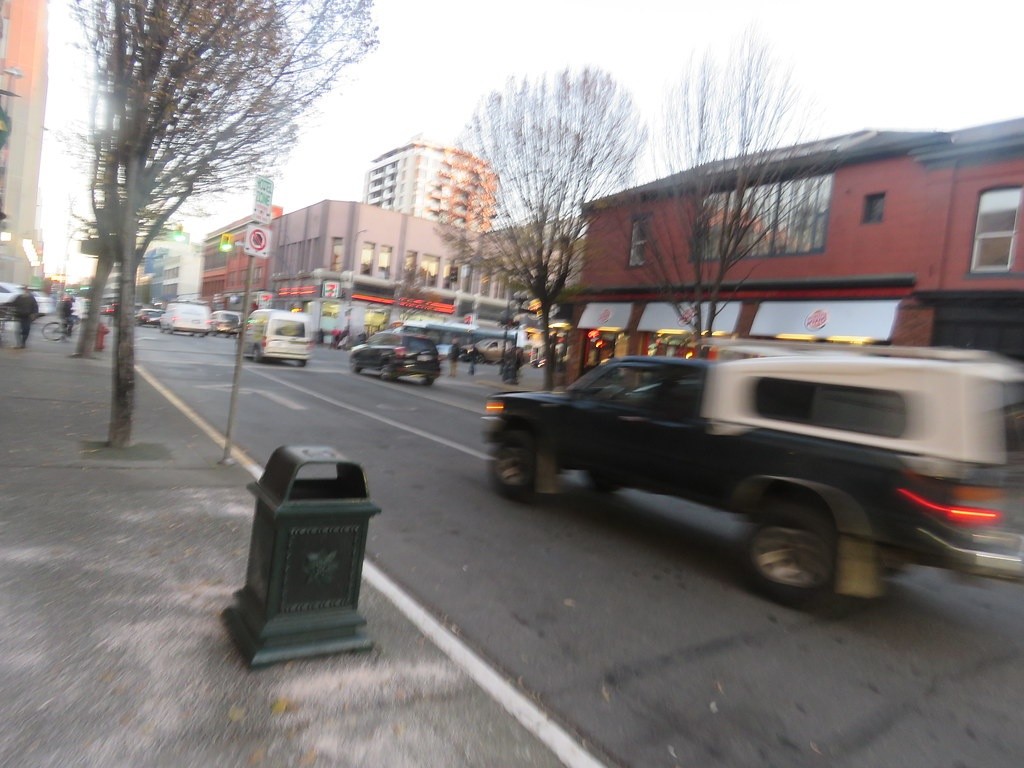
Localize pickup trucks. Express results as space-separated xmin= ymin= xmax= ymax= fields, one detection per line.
xmin=483 ymin=342 xmax=1024 ymax=611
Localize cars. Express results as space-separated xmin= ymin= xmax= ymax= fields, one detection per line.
xmin=71 ymin=297 xmax=161 ymax=328
xmin=0 ymin=282 xmax=53 ymax=320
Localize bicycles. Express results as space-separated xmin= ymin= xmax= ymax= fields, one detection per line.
xmin=42 ymin=317 xmax=82 ymax=342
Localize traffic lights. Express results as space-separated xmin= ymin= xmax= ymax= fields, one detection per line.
xmin=172 ymin=224 xmax=184 ymax=241
xmin=219 ymin=233 xmax=232 ymax=253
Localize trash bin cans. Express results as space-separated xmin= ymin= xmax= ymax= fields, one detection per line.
xmin=219 ymin=444 xmax=382 ymax=669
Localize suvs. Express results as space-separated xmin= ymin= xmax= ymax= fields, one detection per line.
xmin=459 ymin=338 xmax=514 ymax=365
xmin=517 ymin=343 xmax=547 ymax=368
xmin=207 ymin=311 xmax=240 ymax=338
xmin=346 ymin=329 xmax=442 ymax=385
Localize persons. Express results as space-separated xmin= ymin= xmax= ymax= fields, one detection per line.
xmin=328 ymin=325 xmax=348 ymax=348
xmin=447 ymin=339 xmax=460 ymax=377
xmin=468 ymin=344 xmax=478 ymax=375
xmin=354 ymin=330 xmax=366 ymax=345
xmin=2 ymin=284 xmax=38 ymax=348
xmin=56 ymin=297 xmax=76 ymax=336
xmin=499 ymin=347 xmax=524 ymax=384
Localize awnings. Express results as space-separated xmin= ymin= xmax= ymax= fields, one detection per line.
xmin=577 ymin=302 xmax=632 ymax=332
xmin=637 ymin=300 xmax=743 ymax=334
xmin=749 ymin=299 xmax=903 ymax=341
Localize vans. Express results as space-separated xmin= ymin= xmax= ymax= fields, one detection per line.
xmin=159 ymin=302 xmax=212 ymax=338
xmin=237 ymin=310 xmax=311 ymax=367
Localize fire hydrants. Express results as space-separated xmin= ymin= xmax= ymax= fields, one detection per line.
xmin=93 ymin=324 xmax=110 ymax=352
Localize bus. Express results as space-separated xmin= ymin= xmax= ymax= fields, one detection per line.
xmin=399 ymin=321 xmax=514 ymax=362
